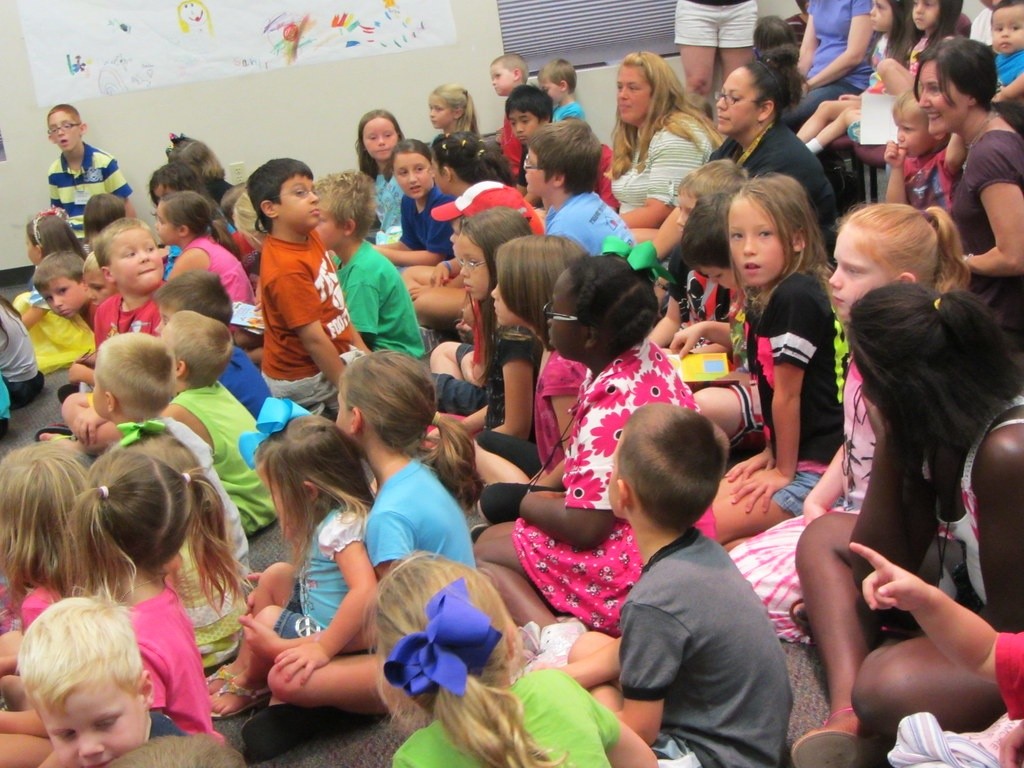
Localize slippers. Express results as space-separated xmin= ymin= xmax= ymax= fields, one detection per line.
xmin=205 ymin=664 xmax=270 ymax=719
xmin=34 ymin=427 xmax=77 ymax=442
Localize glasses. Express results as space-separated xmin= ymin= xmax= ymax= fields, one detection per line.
xmin=454 ymin=256 xmax=487 ymax=271
xmin=48 ymin=123 xmax=81 ymax=135
xmin=542 ymin=298 xmax=577 ymax=323
xmin=713 ymin=91 xmax=760 ymax=106
xmin=265 ymin=189 xmax=317 ymax=200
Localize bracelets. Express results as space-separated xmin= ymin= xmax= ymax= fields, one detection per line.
xmin=961 ymin=253 xmax=973 ymax=263
xmin=441 ymin=261 xmax=454 ymax=273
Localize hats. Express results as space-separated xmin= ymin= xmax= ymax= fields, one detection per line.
xmin=431 ymin=180 xmax=544 ymax=236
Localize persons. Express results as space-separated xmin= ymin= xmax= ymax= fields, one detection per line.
xmin=0 ymin=0 xmax=1024 ymax=768
xmin=16 ymin=597 xmax=192 ymax=768
xmin=203 ymin=416 xmax=379 ymax=719
xmin=531 ymin=404 xmax=795 ymax=768
xmin=602 ymin=49 xmax=721 ymax=229
xmin=246 ymin=158 xmax=375 ymax=412
xmin=46 ymin=104 xmax=137 ymax=255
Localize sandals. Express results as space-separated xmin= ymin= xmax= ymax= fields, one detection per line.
xmin=789 ymin=706 xmax=888 ymax=768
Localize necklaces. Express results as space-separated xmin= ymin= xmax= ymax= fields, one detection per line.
xmin=962 ymin=112 xmax=1000 ymax=173
xmin=119 ymin=575 xmax=160 ymax=604
xmin=923 ymin=462 xmax=963 ymax=601
